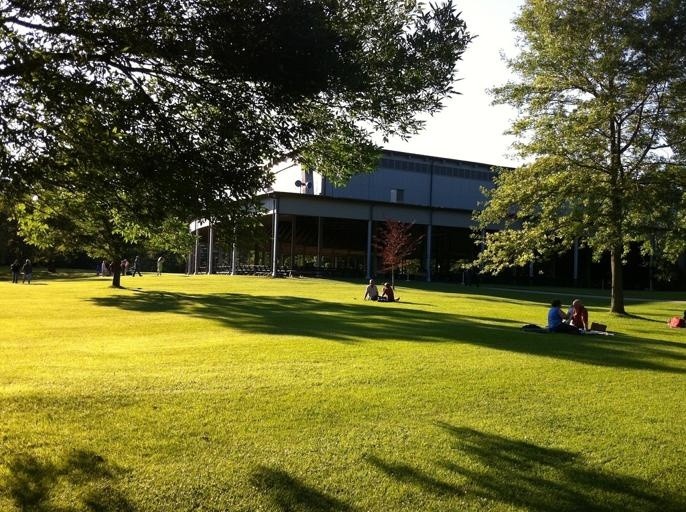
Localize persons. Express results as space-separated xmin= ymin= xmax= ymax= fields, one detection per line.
xmin=156 ymin=256 xmax=165 ymax=276
xmin=377 ymin=282 xmax=395 ymax=302
xmin=547 ymin=299 xmax=583 ymax=334
xmin=563 ymin=297 xmax=580 ymax=325
xmin=9 ymin=259 xmax=20 ymax=283
xmin=95 ymin=256 xmax=144 ymax=278
xmin=362 ymin=278 xmax=401 ymax=302
xmin=569 ymin=298 xmax=591 ymax=332
xmin=22 ymin=258 xmax=33 ymax=284
